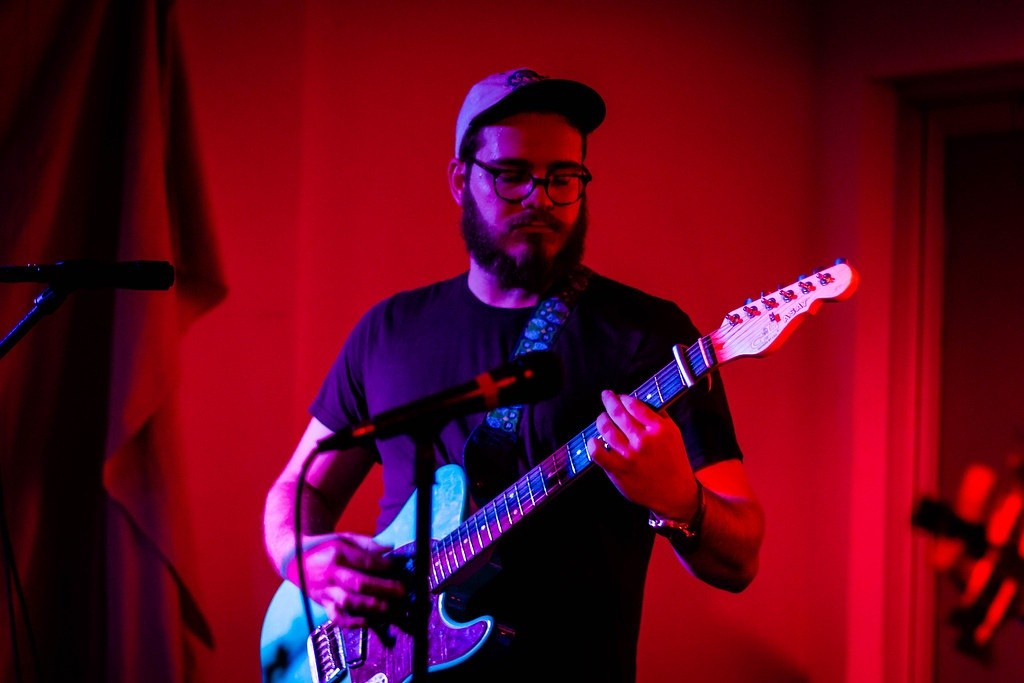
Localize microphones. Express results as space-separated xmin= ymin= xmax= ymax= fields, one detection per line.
xmin=314 ymin=345 xmax=565 ymax=451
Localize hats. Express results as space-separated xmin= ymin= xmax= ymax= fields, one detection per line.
xmin=455 ymin=68 xmax=606 ymax=159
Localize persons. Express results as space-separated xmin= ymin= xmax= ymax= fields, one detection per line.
xmin=263 ymin=70 xmax=765 ymax=682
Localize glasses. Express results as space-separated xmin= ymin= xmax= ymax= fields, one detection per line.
xmin=470 ymin=156 xmax=593 ymax=206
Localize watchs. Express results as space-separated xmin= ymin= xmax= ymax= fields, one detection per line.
xmin=649 ymin=478 xmax=702 ymax=541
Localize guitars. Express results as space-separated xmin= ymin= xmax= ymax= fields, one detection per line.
xmin=257 ymin=256 xmax=862 ymax=683
